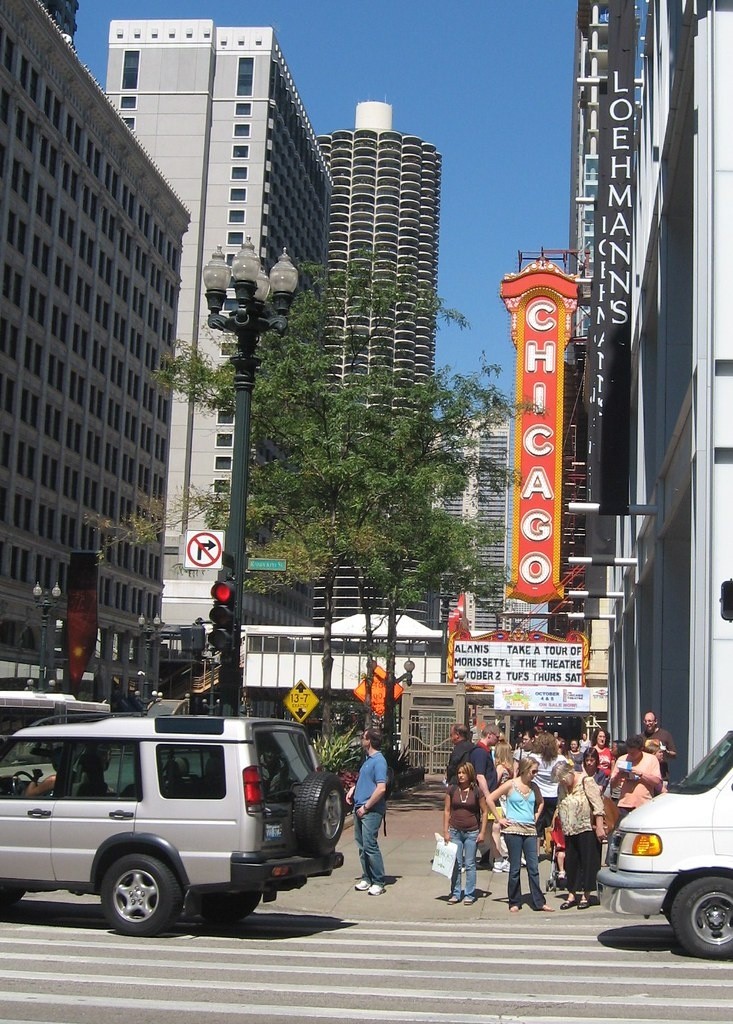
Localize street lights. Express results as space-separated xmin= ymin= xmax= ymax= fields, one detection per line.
xmin=25 ymin=581 xmax=62 ymax=692
xmin=135 ymin=612 xmax=163 ymax=716
xmin=202 ymin=233 xmax=296 ymax=714
xmin=202 ymin=657 xmax=220 ymax=715
xmin=367 ymin=659 xmax=415 ymax=766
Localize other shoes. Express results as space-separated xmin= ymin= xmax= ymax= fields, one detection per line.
xmin=578 ymin=900 xmax=590 ymax=909
xmin=560 ymin=900 xmax=577 ymax=909
xmin=542 ymin=903 xmax=555 ymax=912
xmin=558 ymin=870 xmax=566 ymax=879
xmin=510 ymin=906 xmax=519 ymax=913
xmin=476 ymin=862 xmax=490 ymax=870
xmin=448 ymin=896 xmax=458 ymax=903
xmin=464 ymin=898 xmax=474 ymax=905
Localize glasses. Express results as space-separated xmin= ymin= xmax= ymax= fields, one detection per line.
xmin=492 ymin=732 xmax=499 ymax=739
xmin=531 ymin=769 xmax=539 ymax=775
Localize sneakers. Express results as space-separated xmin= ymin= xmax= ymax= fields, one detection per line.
xmin=492 ymin=860 xmax=510 ymax=872
xmin=355 ymin=880 xmax=372 ymax=891
xmin=368 ymin=885 xmax=385 ymax=896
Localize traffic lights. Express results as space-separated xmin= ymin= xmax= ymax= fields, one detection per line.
xmin=208 ymin=581 xmax=237 ymax=650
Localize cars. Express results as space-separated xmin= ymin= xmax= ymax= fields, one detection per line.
xmin=597 ymin=729 xmax=733 ymax=961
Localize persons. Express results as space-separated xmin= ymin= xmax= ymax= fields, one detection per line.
xmin=443 ymin=761 xmax=488 ymax=905
xmin=485 ymin=757 xmax=556 ymax=912
xmin=24 ymin=747 xmax=63 ymax=797
xmin=550 ymin=763 xmax=608 ymax=910
xmin=345 ymin=728 xmax=388 ymax=896
xmin=445 ymin=711 xmax=677 ymax=879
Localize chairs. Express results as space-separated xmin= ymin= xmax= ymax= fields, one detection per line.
xmin=165 ymin=752 xmax=224 ymax=799
xmin=72 ymin=752 xmax=107 ymax=797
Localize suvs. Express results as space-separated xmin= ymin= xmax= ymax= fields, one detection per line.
xmin=0 ymin=711 xmax=345 ymax=938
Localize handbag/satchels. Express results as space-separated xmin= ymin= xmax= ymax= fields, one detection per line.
xmin=431 ymin=832 xmax=458 ymax=879
xmin=543 ymin=826 xmax=553 ymax=860
xmin=591 ymin=796 xmax=620 ymax=835
xmin=385 ymin=767 xmax=395 ymax=802
